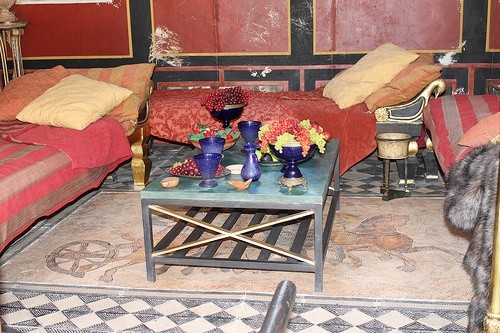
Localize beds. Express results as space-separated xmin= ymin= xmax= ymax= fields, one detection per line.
xmin=0 ymin=61 xmax=157 ymax=254
xmin=424 ymin=92 xmax=500 ymax=333
xmin=146 ymin=53 xmax=446 ymax=164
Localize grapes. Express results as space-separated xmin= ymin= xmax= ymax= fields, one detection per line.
xmin=203 ymin=87 xmax=249 ymax=112
xmin=170 ymin=159 xmax=224 ymax=177
xmin=260 ymin=116 xmax=326 ymax=157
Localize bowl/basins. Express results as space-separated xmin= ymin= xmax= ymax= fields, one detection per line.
xmin=189 ymin=138 xmax=236 ymax=153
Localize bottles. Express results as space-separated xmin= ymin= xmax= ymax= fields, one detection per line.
xmin=240 ymin=143 xmax=262 ymax=182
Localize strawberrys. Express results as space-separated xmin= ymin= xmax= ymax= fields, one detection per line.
xmin=192 ymin=122 xmax=235 ymax=140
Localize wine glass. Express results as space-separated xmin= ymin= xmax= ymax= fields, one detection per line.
xmin=238 ymin=121 xmax=262 ymax=149
xmin=193 ymin=153 xmax=223 ymax=188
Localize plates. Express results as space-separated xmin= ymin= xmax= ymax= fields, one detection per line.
xmin=165 ymin=165 xmax=231 ymax=178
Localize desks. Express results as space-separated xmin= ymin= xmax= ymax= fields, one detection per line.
xmin=140 ymin=139 xmax=342 ymax=293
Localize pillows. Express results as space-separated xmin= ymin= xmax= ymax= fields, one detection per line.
xmin=16 ymin=74 xmax=133 ymax=131
xmin=323 ymin=43 xmax=442 ymax=113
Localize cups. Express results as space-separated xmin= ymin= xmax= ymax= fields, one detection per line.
xmin=199 ymin=136 xmax=225 ymax=154
